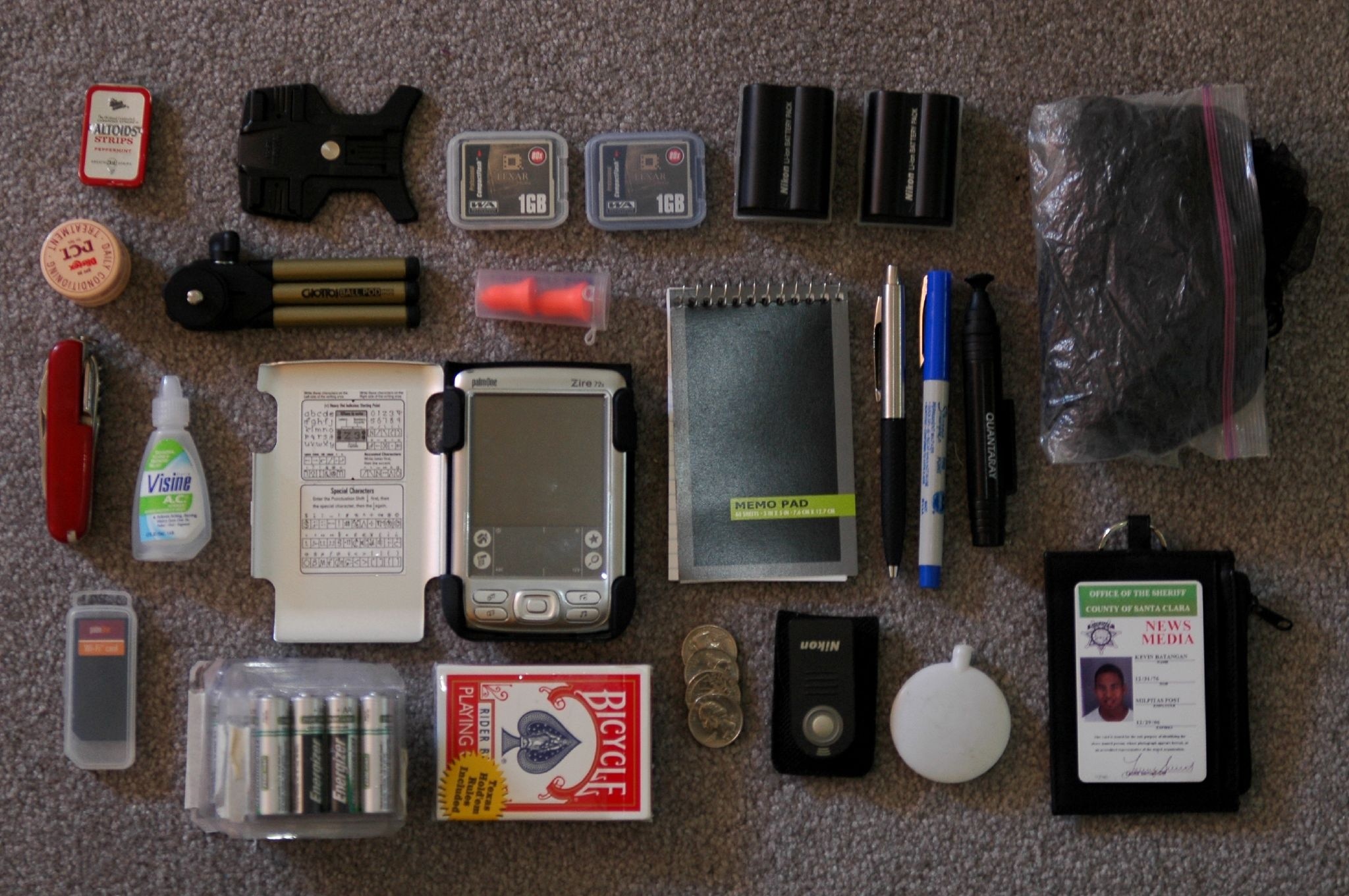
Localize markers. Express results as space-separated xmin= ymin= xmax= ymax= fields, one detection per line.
xmin=964 ymin=272 xmax=1019 ymax=547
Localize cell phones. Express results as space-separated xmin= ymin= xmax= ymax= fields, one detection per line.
xmin=448 ymin=364 xmax=631 ymax=635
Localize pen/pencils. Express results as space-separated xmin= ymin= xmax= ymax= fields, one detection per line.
xmin=872 ymin=265 xmax=909 ymax=579
xmin=917 ymin=270 xmax=954 ymax=589
